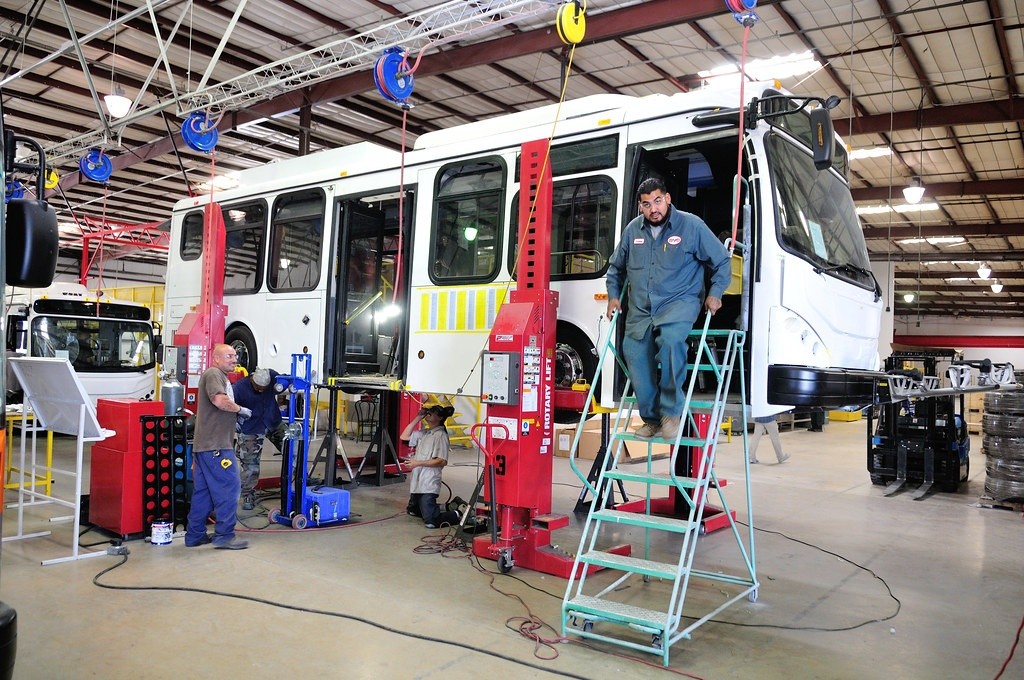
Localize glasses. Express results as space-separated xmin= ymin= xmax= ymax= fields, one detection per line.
xmin=224 ymin=354 xmax=239 ymax=360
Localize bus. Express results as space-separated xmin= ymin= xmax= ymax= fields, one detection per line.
xmin=160 ymin=79 xmax=887 ymax=425
xmin=1 ymin=114 xmax=59 ymax=680
xmin=5 ymin=281 xmax=162 ymax=428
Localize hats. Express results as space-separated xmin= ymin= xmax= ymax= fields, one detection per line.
xmin=252 ymin=366 xmax=270 ymax=386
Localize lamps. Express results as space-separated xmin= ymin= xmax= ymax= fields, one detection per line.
xmin=902 ymin=181 xmax=925 ymax=203
xmin=104 ymin=84 xmax=132 ymax=117
xmin=976 ymin=262 xmax=1003 ymax=293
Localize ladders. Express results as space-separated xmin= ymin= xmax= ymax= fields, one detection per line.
xmin=561 ymin=174 xmax=761 ymax=668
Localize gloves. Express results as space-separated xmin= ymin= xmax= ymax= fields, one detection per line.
xmin=237 ymin=406 xmax=253 ymax=425
xmin=236 ymin=422 xmax=241 ymax=432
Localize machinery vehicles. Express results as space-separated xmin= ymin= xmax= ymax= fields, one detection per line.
xmin=866 ymin=345 xmax=970 ymax=501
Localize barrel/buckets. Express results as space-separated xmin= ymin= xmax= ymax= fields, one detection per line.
xmin=151 ymin=518 xmax=174 ymax=546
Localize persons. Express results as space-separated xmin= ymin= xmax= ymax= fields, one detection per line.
xmin=184 ymin=344 xmax=253 ymax=549
xmin=232 ymin=368 xmax=291 ymax=510
xmin=606 ymin=178 xmax=732 ymax=440
xmin=399 ymin=405 xmax=477 ymax=529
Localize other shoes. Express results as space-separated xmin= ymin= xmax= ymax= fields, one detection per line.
xmin=778 ymin=451 xmax=790 ymax=464
xmin=633 ymin=422 xmax=660 ymax=440
xmin=749 ymin=459 xmax=760 ymax=464
xmin=450 ymin=496 xmax=475 ymax=523
xmin=241 ymin=494 xmax=254 ymax=510
xmin=660 ymin=415 xmax=680 ymax=440
xmin=185 ymin=533 xmax=248 ymax=550
